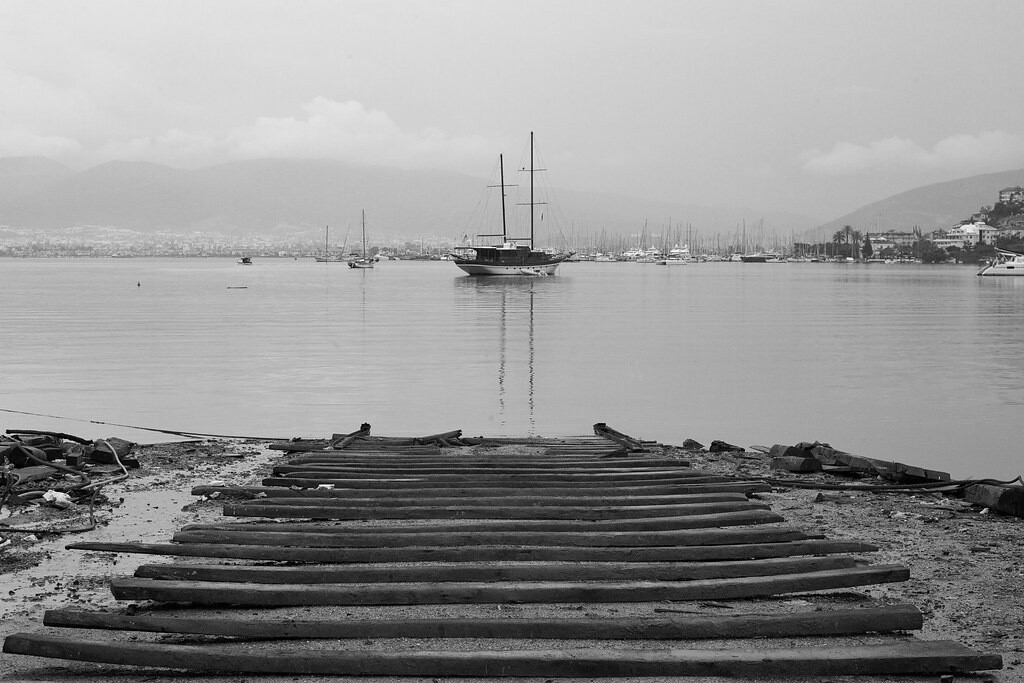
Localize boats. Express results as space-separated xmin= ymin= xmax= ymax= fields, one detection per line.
xmin=977 ymin=252 xmax=1024 ymax=276
xmin=885 ymin=259 xmax=894 ymax=264
xmin=237 ymin=257 xmax=252 ymax=265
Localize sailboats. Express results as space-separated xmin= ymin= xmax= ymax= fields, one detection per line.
xmin=720 ymin=219 xmax=819 ymax=263
xmin=565 ymin=217 xmax=707 ymax=266
xmin=347 ymin=209 xmax=375 ymax=268
xmin=449 ymin=131 xmax=567 ymax=276
xmin=315 ymin=225 xmax=342 ymax=262
